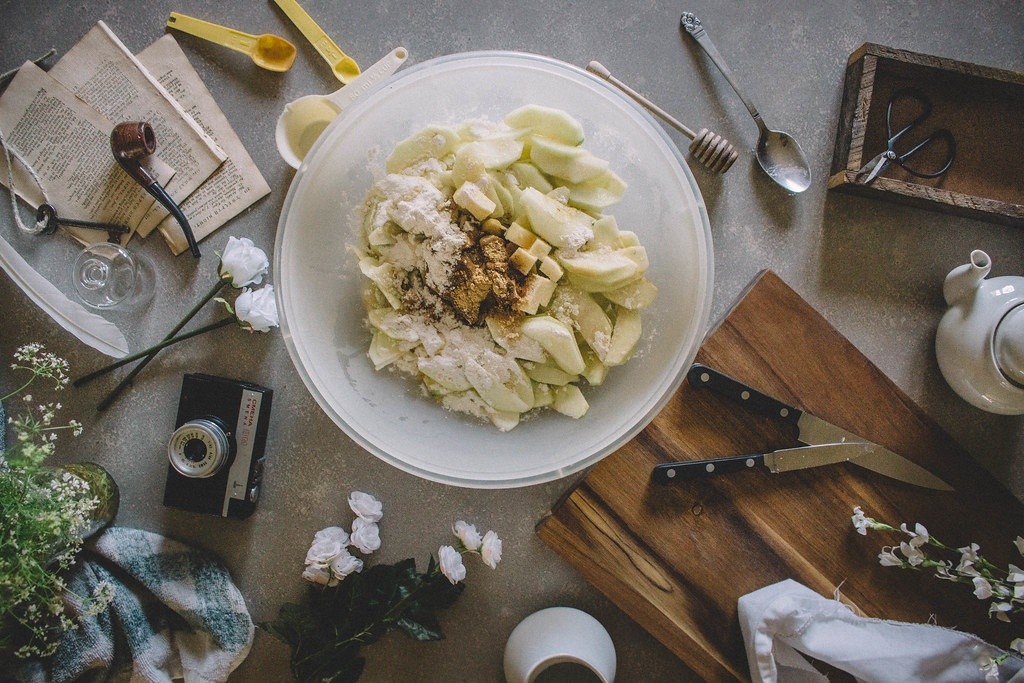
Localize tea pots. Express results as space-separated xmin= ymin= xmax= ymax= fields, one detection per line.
xmin=934 ymin=249 xmax=1024 ymax=417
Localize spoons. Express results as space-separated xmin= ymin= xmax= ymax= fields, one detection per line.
xmin=681 ymin=13 xmax=812 ymax=193
xmin=165 ymin=10 xmax=296 ymax=71
xmin=272 ymin=1 xmax=360 ymax=84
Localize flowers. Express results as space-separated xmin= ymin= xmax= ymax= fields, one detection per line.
xmin=851 ymin=506 xmax=1023 ymax=657
xmin=69 ymin=236 xmax=280 ymax=417
xmin=284 ymin=487 xmax=504 ymax=682
xmin=0 ymin=341 xmax=112 ymax=659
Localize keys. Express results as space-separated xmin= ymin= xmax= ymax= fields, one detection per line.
xmin=34 ymin=204 xmax=130 ymax=247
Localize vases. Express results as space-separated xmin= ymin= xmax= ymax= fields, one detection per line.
xmin=53 ymin=456 xmax=123 ymax=545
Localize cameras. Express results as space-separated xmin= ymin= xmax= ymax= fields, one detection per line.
xmin=163 ymin=373 xmax=274 ymax=520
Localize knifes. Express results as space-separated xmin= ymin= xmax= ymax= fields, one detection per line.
xmin=650 ymin=441 xmax=882 ymax=484
xmin=685 ymin=360 xmax=953 ymax=490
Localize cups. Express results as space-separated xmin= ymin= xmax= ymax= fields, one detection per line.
xmin=73 ymin=243 xmax=155 ymax=313
xmin=503 ymin=608 xmax=618 ymax=682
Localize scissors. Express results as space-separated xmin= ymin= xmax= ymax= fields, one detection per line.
xmin=858 ymin=92 xmax=956 ymax=184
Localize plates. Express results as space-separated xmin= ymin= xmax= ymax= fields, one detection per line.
xmin=274 ymin=51 xmax=714 ymax=492
